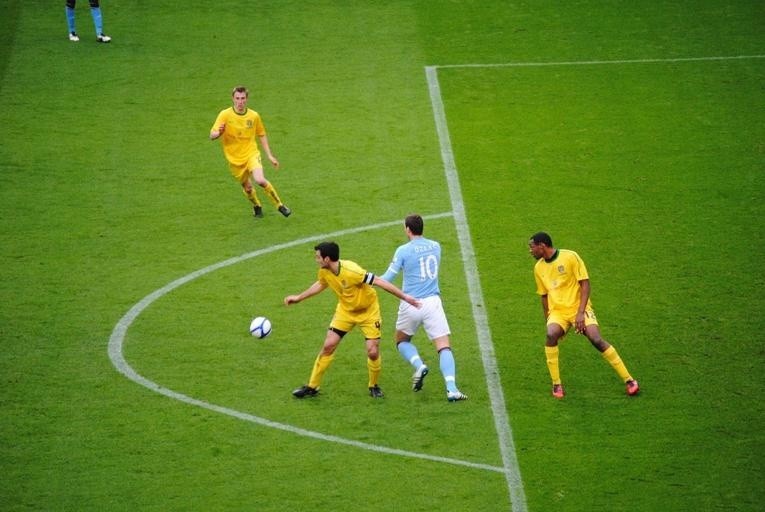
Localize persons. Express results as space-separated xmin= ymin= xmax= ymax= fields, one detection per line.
xmin=378 ymin=214 xmax=469 ymax=403
xmin=528 ymin=233 xmax=640 ymax=400
xmin=65 ymin=0 xmax=112 ymax=44
xmin=209 ymin=85 xmax=292 ymax=220
xmin=283 ymin=242 xmax=422 ymax=399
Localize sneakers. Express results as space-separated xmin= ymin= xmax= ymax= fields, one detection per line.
xmin=369 ymin=384 xmax=385 ymax=399
xmin=253 ymin=205 xmax=264 ymax=218
xmin=95 ymin=33 xmax=112 ymax=43
xmin=69 ymin=32 xmax=79 ymax=41
xmin=411 ymin=365 xmax=429 ymax=392
xmin=626 ymin=378 xmax=639 ymax=396
xmin=277 ymin=203 xmax=291 ymax=218
xmin=552 ymin=383 xmax=565 ymax=399
xmin=292 ymin=384 xmax=320 ymax=398
xmin=447 ymin=390 xmax=468 ymax=402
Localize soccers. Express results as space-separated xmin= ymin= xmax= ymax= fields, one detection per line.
xmin=250 ymin=315 xmax=272 ymax=338
